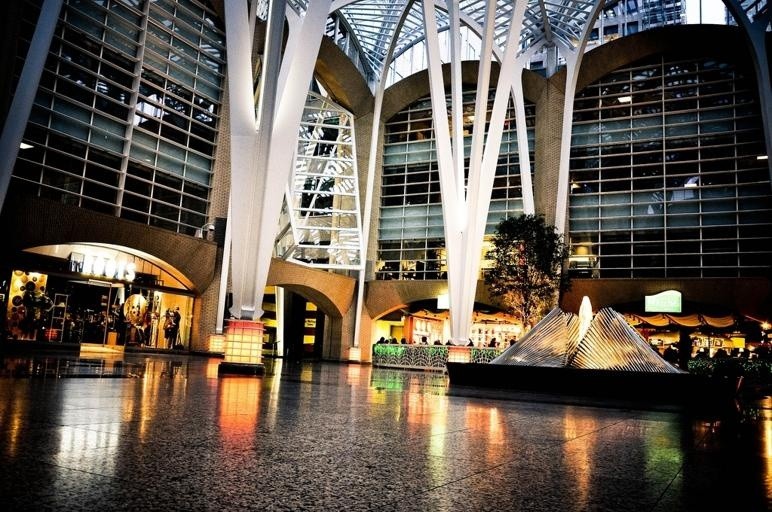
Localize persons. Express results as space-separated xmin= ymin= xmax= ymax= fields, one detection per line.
xmin=163 ymin=307 xmax=171 ymax=348
xmin=168 ymin=306 xmax=182 ymax=349
xmin=374 ymin=336 xmax=516 ymax=369
xmin=23 ymin=281 xmax=36 ymax=340
xmin=36 ymin=295 xmax=55 ymax=341
xmin=168 ymin=312 xmax=177 ymax=328
